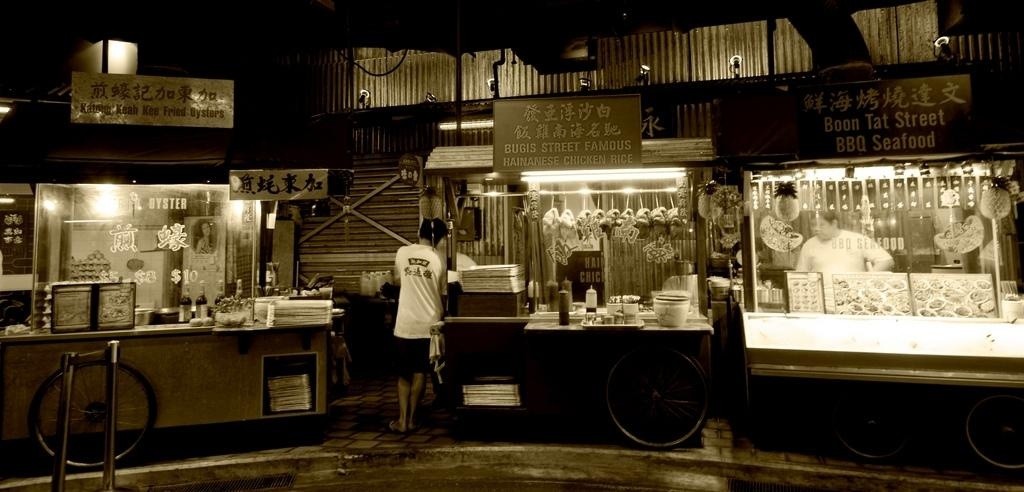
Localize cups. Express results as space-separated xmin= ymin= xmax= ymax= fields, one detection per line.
xmin=584 ymin=310 xmax=637 ymax=324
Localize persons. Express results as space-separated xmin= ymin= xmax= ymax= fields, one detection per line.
xmin=387 ymin=217 xmax=448 ymax=432
xmin=795 ymin=209 xmax=895 ymax=274
xmin=195 ymin=221 xmax=212 ymax=254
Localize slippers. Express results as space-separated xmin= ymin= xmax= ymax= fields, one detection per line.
xmin=383 ymin=420 xmax=407 ymax=434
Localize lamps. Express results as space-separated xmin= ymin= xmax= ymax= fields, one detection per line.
xmin=635 ymin=64 xmax=651 ymax=86
xmin=933 ymin=35 xmax=954 ymax=61
xmin=490 ymin=80 xmax=496 ymax=93
xmin=358 ymin=90 xmax=370 ymax=109
xmin=423 ymin=92 xmax=437 ymax=103
xmin=580 ymin=71 xmax=592 ymax=92
xmin=729 ymin=55 xmax=743 ymax=77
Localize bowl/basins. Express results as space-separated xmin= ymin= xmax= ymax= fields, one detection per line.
xmin=135 ymin=308 xmax=179 ymax=325
xmin=1002 ymin=299 xmax=1024 ymax=322
xmin=673 ymin=259 xmax=698 ymax=275
xmin=707 ymin=279 xmax=731 ymax=301
xmin=758 ymin=288 xmax=786 ymax=312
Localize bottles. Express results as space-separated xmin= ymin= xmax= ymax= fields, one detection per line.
xmin=359 ymin=270 xmax=392 ymax=297
xmin=560 ymin=275 xmax=573 ymax=311
xmin=195 ymin=279 xmax=207 ymax=319
xmin=214 ymin=279 xmax=225 ymax=306
xmin=527 ymin=280 xmax=539 ymax=314
xmin=234 ymin=278 xmax=245 ymax=300
xmin=178 ymin=280 xmax=191 ymax=325
xmin=557 ymin=287 xmax=569 ymax=325
xmin=545 ymin=279 xmax=558 ymax=311
xmin=584 ymin=284 xmax=597 ymax=312
